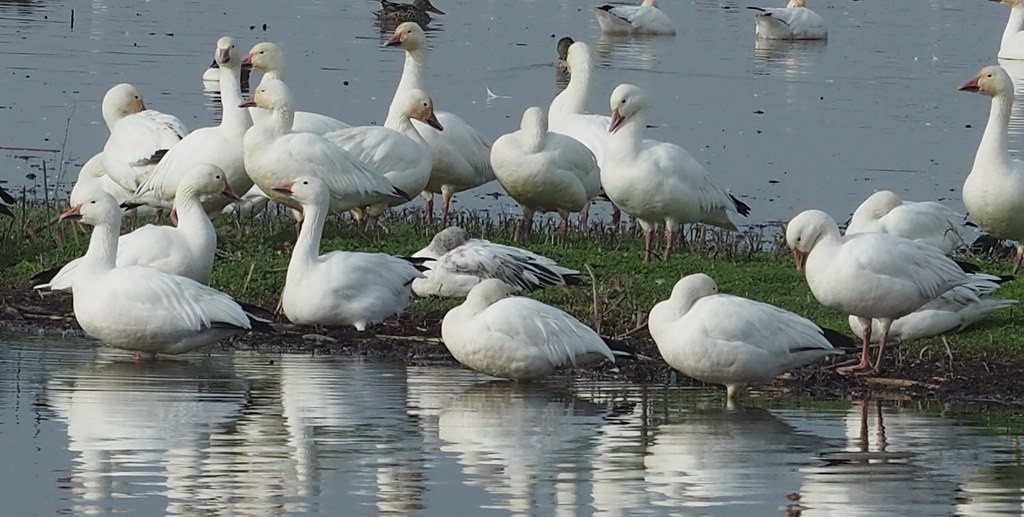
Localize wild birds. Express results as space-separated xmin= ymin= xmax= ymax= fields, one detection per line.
xmin=31 ymin=0 xmax=1024 ymax=404
